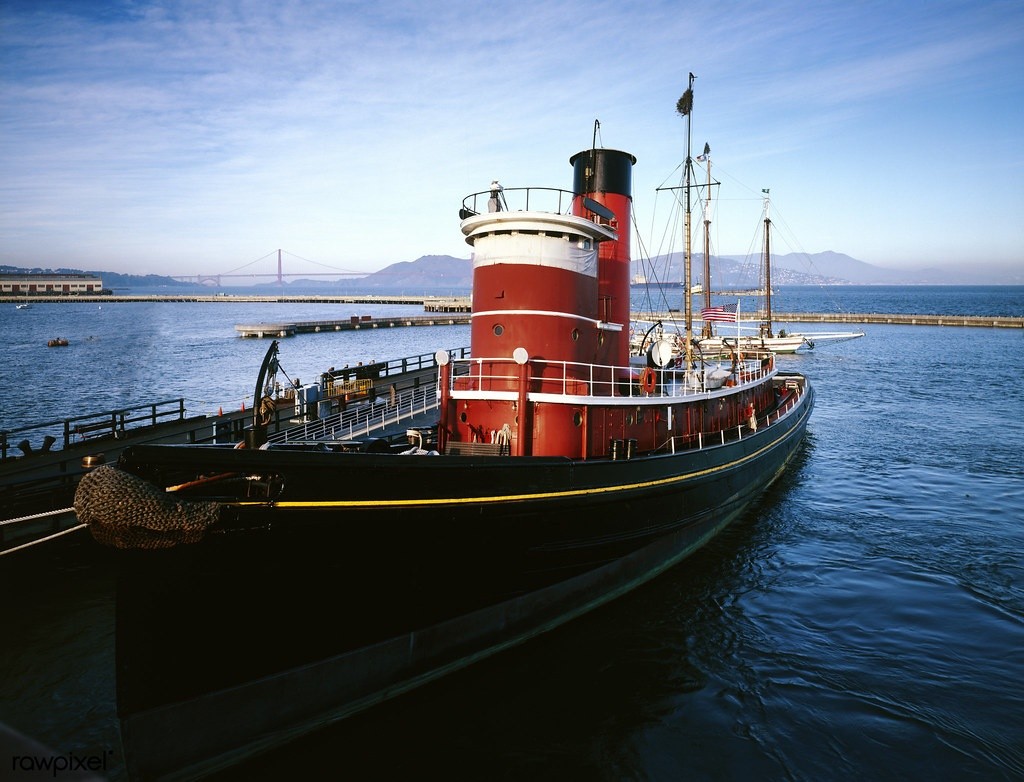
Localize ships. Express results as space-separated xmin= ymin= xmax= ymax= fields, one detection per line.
xmin=71 ymin=67 xmax=819 ymax=782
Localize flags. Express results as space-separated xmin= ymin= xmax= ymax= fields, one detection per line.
xmin=696 ymin=154 xmax=707 ymax=162
xmin=700 ymin=303 xmax=738 ymax=323
xmin=761 ymin=189 xmax=770 ymax=194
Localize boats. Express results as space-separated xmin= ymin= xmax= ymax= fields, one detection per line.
xmin=626 ymin=190 xmax=865 ymax=355
xmin=16 ymin=303 xmax=31 ymax=309
xmin=687 ymin=364 xmax=733 ymax=389
xmin=47 ymin=337 xmax=68 ymax=347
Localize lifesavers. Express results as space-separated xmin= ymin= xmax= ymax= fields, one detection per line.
xmin=743 ymin=403 xmax=753 ymax=420
xmin=643 ymin=368 xmax=657 ymax=394
xmin=769 ymin=356 xmax=773 ymax=371
